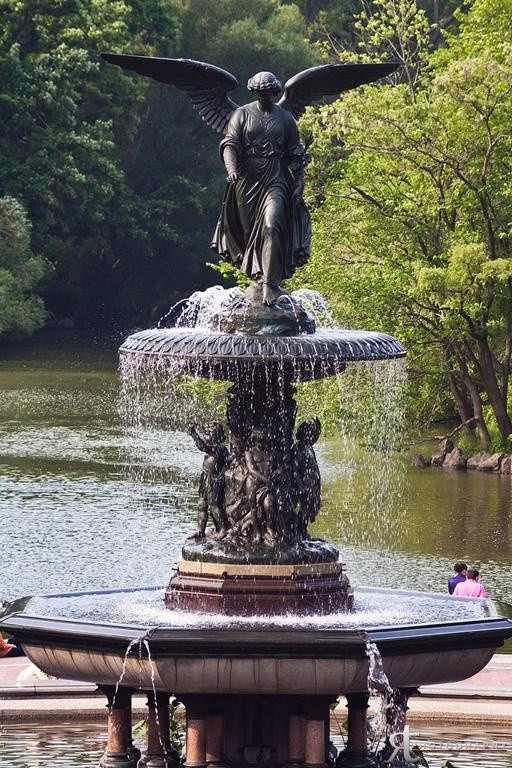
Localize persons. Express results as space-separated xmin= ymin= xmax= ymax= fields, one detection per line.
xmin=447 ymin=560 xmax=468 ymax=594
xmin=0 ymin=632 xmax=27 ymax=658
xmin=99 ymin=51 xmax=411 ymax=305
xmin=450 ymin=569 xmax=487 ymax=599
xmin=186 ymin=415 xmax=322 ymax=549
